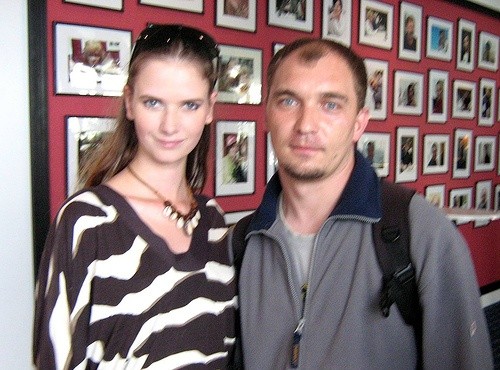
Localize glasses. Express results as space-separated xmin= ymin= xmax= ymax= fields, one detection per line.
xmin=129 ymin=25 xmax=220 ymax=87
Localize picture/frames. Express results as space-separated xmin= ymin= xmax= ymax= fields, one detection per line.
xmin=452 ymin=129 xmax=500 ymax=179
xmin=363 ymin=59 xmax=391 ymax=122
xmin=395 ymin=126 xmax=421 ymax=184
xmin=392 ymin=70 xmax=425 ymax=116
xmin=423 ymin=180 xmax=500 ymax=228
xmin=398 ymin=2 xmax=424 ymax=63
xmin=457 ymin=17 xmax=476 ymax=73
xmin=478 ymin=77 xmax=499 ymax=127
xmin=320 ymin=0 xmax=354 ymax=48
xmin=450 ymin=79 xmax=477 ymax=120
xmin=422 ymin=133 xmax=451 ymax=175
xmin=478 ymin=31 xmax=499 ymax=73
xmin=53 ymin=0 xmax=315 ymax=228
xmin=426 ymin=16 xmax=454 ymax=62
xmin=359 ymin=0 xmax=394 ymax=52
xmin=426 ymin=69 xmax=450 ymax=124
xmin=357 ymin=132 xmax=392 ymax=178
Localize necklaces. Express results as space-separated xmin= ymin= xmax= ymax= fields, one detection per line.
xmin=126 ymin=163 xmax=201 ymax=237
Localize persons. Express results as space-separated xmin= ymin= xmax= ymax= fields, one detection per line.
xmin=461 ymin=32 xmax=472 ymax=64
xmin=437 ymin=29 xmax=447 ymax=53
xmin=456 ymin=138 xmax=466 ymax=169
xmin=365 ymin=142 xmax=376 ymax=168
xmin=433 ymin=80 xmax=443 ymax=113
xmin=31 ymin=24 xmax=246 ymax=370
xmin=482 ymin=87 xmax=492 ymax=118
xmin=427 ymin=143 xmax=438 ymax=166
xmin=222 ymin=132 xmax=248 ymax=184
xmin=327 ymin=1 xmax=347 ymax=37
xmin=482 ymin=41 xmax=495 ymax=63
xmin=226 ymin=38 xmax=495 ymax=370
xmin=364 ymin=9 xmax=386 ymax=35
xmin=406 ymin=84 xmax=417 ymax=106
xmin=404 ymin=16 xmax=417 ymax=51
